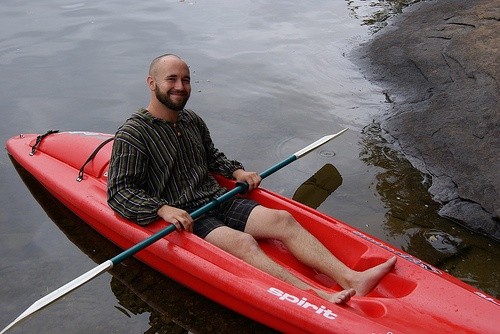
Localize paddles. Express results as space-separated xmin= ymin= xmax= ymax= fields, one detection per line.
xmin=0 ymin=127 xmax=350 ymax=334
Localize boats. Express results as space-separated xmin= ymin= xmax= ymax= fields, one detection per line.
xmin=6 ymin=129 xmax=500 ymax=334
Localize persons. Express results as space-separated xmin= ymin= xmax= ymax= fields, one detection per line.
xmin=107 ymin=54 xmax=397 ymax=304
